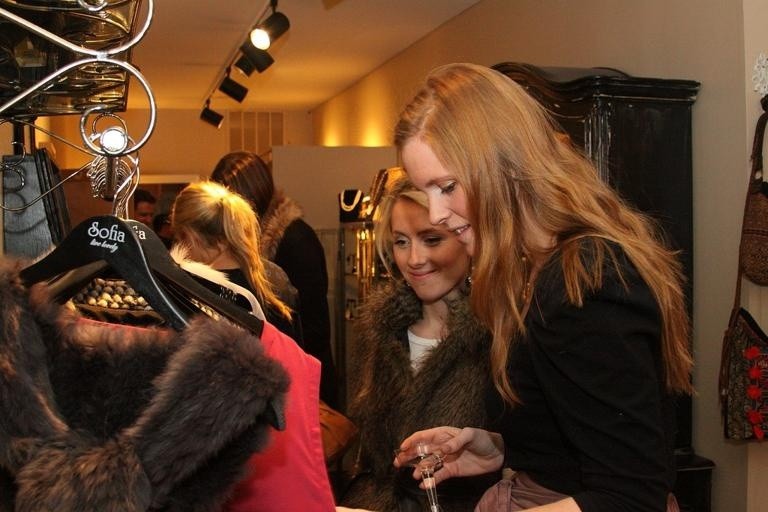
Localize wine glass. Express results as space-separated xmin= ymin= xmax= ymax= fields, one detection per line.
xmin=394 ymin=436 xmax=447 ymax=511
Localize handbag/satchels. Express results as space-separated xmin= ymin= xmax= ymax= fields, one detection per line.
xmin=468 ymin=472 xmax=681 ymax=512
xmin=719 ymin=307 xmax=768 ymax=441
xmin=319 ymin=400 xmax=359 ymax=466
xmin=739 ymin=178 xmax=768 ymax=286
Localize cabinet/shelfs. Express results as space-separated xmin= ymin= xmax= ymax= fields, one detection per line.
xmin=335 ymin=221 xmax=376 ymax=412
xmin=490 ymin=62 xmax=716 ymax=512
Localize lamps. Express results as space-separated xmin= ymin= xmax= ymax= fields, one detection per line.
xmin=200 ymin=0 xmax=290 ymax=128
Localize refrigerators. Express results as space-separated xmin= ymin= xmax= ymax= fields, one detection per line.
xmin=337 ymin=189 xmax=379 ymax=463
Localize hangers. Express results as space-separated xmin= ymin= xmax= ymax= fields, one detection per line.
xmin=19 ymin=132 xmax=287 ymax=432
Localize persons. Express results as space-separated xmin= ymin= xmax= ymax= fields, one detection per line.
xmin=394 ymin=63 xmax=699 ymax=512
xmin=170 ymin=181 xmax=332 ymax=403
xmin=133 ymin=189 xmax=180 ymax=252
xmin=335 ymin=176 xmax=504 ymax=512
xmin=210 ymin=151 xmax=339 ymax=412
